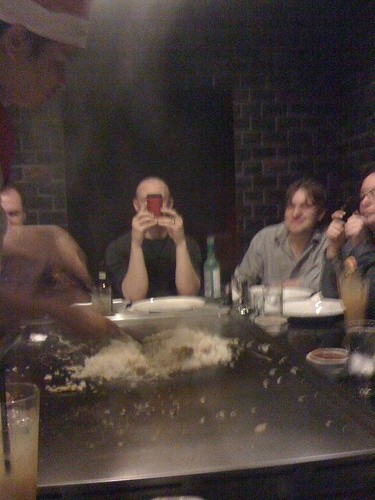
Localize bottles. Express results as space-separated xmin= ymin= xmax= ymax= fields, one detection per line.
xmin=95 ymin=272 xmax=113 ymax=315
xmin=202 ymin=243 xmax=220 ymax=302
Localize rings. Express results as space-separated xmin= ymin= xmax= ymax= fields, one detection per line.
xmin=174 ymin=219 xmax=175 ymax=224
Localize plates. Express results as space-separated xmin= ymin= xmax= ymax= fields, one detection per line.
xmin=283 ymin=297 xmax=342 ymax=318
xmin=130 ymin=295 xmax=205 ymax=312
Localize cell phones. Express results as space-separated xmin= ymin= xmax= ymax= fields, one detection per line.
xmin=342 ymin=196 xmax=359 ymax=223
xmin=147 ymin=194 xmax=163 ymax=217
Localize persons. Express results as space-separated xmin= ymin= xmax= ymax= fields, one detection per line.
xmin=0 ymin=0 xmax=121 ymax=341
xmin=104 ymin=175 xmax=204 ymax=302
xmin=321 ymin=166 xmax=375 ymax=319
xmin=226 ymin=181 xmax=329 ymax=292
xmin=0 ymin=184 xmax=27 ymax=225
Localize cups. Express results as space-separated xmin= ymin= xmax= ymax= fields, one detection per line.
xmin=346 ymin=318 xmax=375 ymax=400
xmin=0 ymin=381 xmax=40 ymax=500
xmin=231 ymin=275 xmax=249 ymax=311
xmin=262 ymin=278 xmax=283 ymax=316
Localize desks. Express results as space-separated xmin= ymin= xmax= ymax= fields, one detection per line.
xmin=0 ymin=304 xmax=375 ymax=500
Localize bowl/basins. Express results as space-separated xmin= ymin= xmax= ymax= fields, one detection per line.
xmin=305 ymin=347 xmax=350 ymax=379
xmin=250 ymin=285 xmax=314 ymax=311
xmin=253 ymin=314 xmax=289 ymax=339
